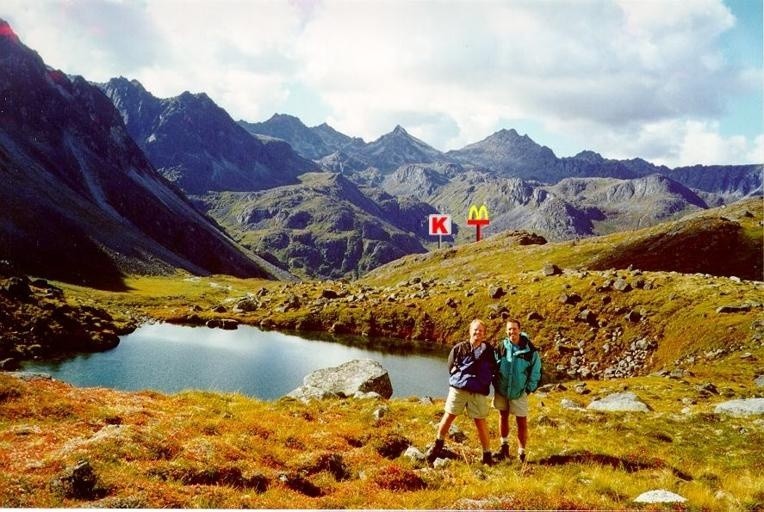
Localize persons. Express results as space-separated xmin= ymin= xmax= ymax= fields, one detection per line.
xmin=489 ymin=318 xmax=541 ymax=463
xmin=417 ymin=318 xmax=498 ymax=468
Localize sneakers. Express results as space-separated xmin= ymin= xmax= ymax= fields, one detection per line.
xmin=427 ymin=439 xmax=444 ymax=458
xmin=519 ymin=453 xmax=527 ymax=464
xmin=493 ymin=444 xmax=510 ymax=459
xmin=482 ymin=451 xmax=495 ymax=467
